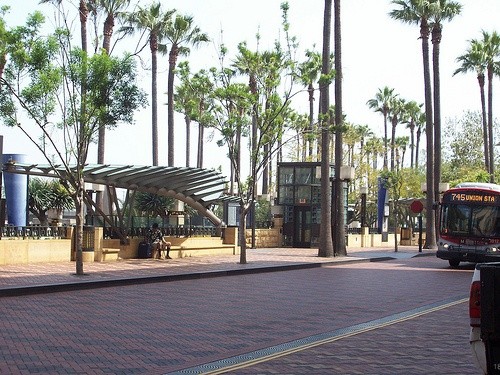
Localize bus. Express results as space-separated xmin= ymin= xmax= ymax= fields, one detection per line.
xmin=432 ymin=182 xmax=500 ymax=267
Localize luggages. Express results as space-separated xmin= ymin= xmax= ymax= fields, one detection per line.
xmin=139 ymin=229 xmax=152 ymax=258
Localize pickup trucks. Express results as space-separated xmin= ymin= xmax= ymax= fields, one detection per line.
xmin=469 ymin=262 xmax=500 ymax=375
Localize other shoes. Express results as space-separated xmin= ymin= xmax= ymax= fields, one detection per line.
xmin=158 ymin=257 xmax=164 ymax=260
xmin=165 ymin=256 xmax=172 ymax=259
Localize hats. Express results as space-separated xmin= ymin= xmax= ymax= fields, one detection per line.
xmin=152 ymin=223 xmax=158 ymax=228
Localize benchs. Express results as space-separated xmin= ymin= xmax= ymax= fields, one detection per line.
xmin=151 ymin=243 xmax=235 ymax=260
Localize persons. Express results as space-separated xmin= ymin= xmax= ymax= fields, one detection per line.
xmin=148 ymin=223 xmax=172 ymax=260
